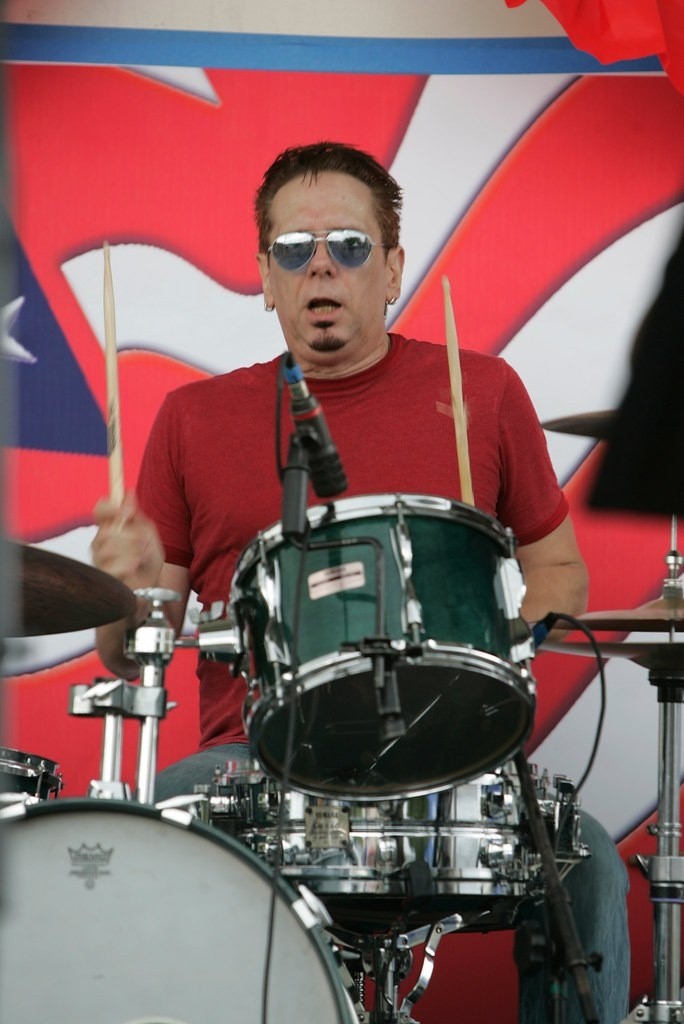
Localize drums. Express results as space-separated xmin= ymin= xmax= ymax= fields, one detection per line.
xmin=194 ymin=771 xmax=590 ymax=935
xmin=228 ymin=493 xmax=539 ymax=802
xmin=1 ymin=796 xmax=366 ymax=1024
xmin=1 ymin=748 xmax=62 ymax=812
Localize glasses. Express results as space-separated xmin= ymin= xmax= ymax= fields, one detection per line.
xmin=266 ymin=228 xmax=390 ymax=272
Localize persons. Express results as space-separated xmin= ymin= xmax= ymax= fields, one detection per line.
xmin=85 ymin=144 xmax=633 ymax=1024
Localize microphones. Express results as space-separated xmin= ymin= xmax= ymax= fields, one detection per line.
xmin=281 ymin=350 xmax=349 ymax=498
xmin=530 ymin=610 xmax=558 ymax=647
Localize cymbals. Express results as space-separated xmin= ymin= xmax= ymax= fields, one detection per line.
xmin=0 ymin=540 xmax=139 ymax=640
xmin=540 ymin=586 xmax=683 ymax=675
